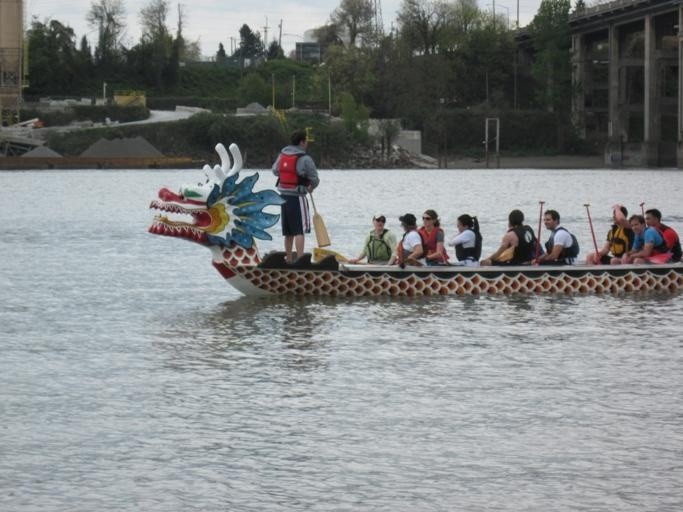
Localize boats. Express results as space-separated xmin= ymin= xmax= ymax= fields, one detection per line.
xmin=149 ymin=143 xmax=682 ymax=297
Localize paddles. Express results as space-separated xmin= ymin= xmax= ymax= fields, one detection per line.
xmin=492 ymin=245 xmax=515 ymax=263
xmin=394 ymin=258 xmax=423 ymax=266
xmin=313 ymin=249 xmax=368 ymax=266
xmin=632 ymin=254 xmax=671 ymax=264
xmin=309 ymin=192 xmax=330 ymax=247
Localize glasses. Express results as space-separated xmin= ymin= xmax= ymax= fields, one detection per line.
xmin=375 ymin=218 xmax=384 ymax=223
xmin=422 ymin=216 xmax=431 ymax=221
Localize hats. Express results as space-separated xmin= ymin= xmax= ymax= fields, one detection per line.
xmin=399 ymin=213 xmax=416 ymax=225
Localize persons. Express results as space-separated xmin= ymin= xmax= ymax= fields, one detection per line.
xmin=272 ymin=128 xmax=319 ymax=265
